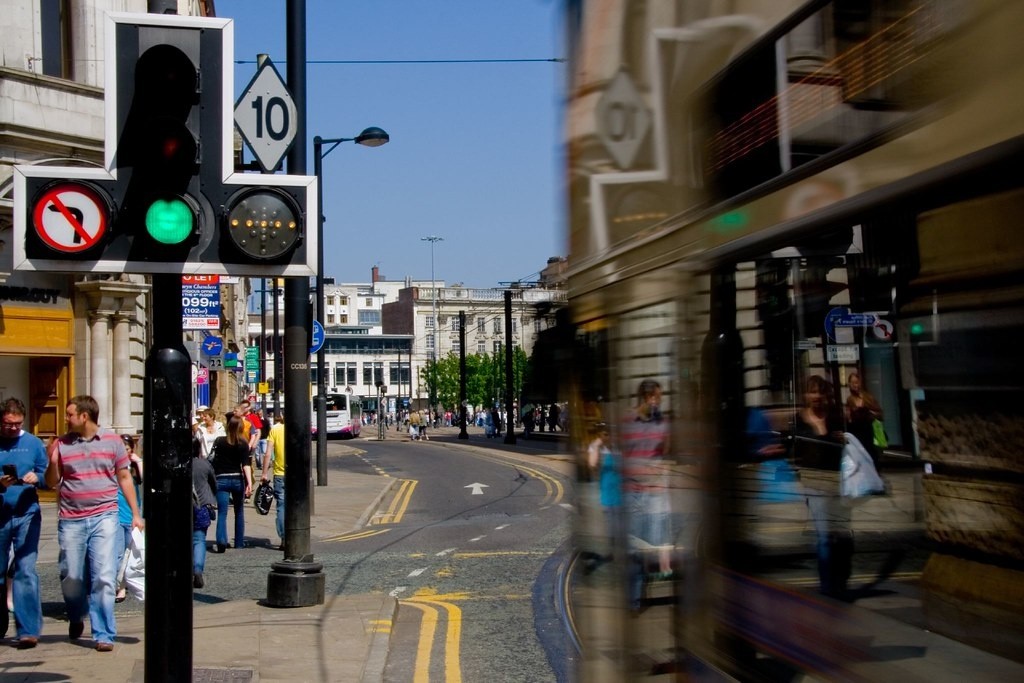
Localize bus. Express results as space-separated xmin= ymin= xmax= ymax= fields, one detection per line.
xmin=311 ymin=392 xmax=365 ymax=437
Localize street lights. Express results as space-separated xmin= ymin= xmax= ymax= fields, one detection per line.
xmin=313 ymin=125 xmax=393 ymax=487
xmin=375 ymin=380 xmax=383 ymax=440
xmin=770 ymin=245 xmax=809 ymax=405
xmin=419 ymin=235 xmax=445 ymax=367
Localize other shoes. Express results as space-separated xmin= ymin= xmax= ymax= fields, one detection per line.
xmin=278 ymin=544 xmax=287 ymax=551
xmin=194 ymin=570 xmax=205 ymax=588
xmin=18 ymin=634 xmax=38 ymax=646
xmin=68 ymin=617 xmax=84 ymax=640
xmin=98 ymin=640 xmax=113 ymax=653
xmin=116 ymin=586 xmax=127 ymax=605
xmin=218 ymin=542 xmax=228 ymax=554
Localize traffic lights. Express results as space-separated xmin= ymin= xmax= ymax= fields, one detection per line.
xmin=12 ymin=167 xmax=117 ymax=273
xmin=222 ymin=172 xmax=324 ymax=280
xmin=102 ymin=16 xmax=234 ymax=275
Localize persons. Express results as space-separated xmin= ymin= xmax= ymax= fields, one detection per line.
xmin=0 ymin=398 xmax=50 ymax=646
xmin=191 ymin=400 xmax=285 ymax=588
xmin=44 ymin=395 xmax=145 ymax=650
xmin=361 ymin=412 xmax=393 ymax=429
xmin=589 ymin=373 xmax=891 ymax=626
xmin=396 ymin=409 xmax=440 ymax=441
xmin=475 ymin=409 xmax=507 ymax=439
xmin=444 ymin=409 xmax=471 ymax=427
xmin=522 ymin=402 xmax=570 ymax=433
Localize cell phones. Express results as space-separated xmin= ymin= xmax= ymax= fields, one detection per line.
xmin=2 ymin=465 xmax=20 ymax=486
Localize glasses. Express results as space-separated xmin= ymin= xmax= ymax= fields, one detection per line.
xmin=0 ymin=422 xmax=23 ymax=429
xmin=123 ymin=437 xmax=131 ymax=441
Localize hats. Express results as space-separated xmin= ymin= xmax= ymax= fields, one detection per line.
xmin=197 ymin=404 xmax=208 ymax=412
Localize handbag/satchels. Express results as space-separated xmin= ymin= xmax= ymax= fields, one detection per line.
xmin=254 ymin=481 xmax=274 ymax=515
xmin=116 ymin=524 xmax=145 ymax=603
xmin=193 ymin=506 xmax=211 ymax=529
